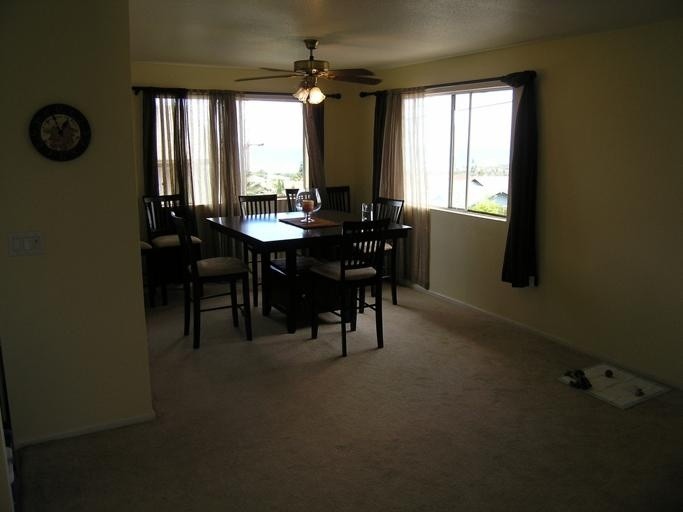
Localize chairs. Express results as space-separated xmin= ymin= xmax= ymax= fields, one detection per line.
xmin=309 ymin=218 xmax=384 ymax=357
xmin=239 ymin=194 xmax=277 ymax=305
xmin=146 ymin=192 xmax=202 ymax=309
xmin=285 ymin=189 xmax=301 ymax=212
xmin=318 ymin=185 xmax=353 ymax=210
xmin=173 ymin=212 xmax=253 ymax=348
xmin=352 ymin=196 xmax=405 ymax=303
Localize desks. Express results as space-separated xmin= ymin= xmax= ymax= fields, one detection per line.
xmin=207 ymin=208 xmax=414 ymax=344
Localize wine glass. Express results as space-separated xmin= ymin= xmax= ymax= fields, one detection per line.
xmin=295 ymin=188 xmax=322 ymax=225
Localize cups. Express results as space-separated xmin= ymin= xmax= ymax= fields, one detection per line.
xmin=362 ymin=201 xmax=379 ymax=227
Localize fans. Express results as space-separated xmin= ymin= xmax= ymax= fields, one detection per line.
xmin=232 ymin=38 xmax=383 ymax=87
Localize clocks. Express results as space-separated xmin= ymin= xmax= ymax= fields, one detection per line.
xmin=28 ymin=102 xmax=93 ymax=161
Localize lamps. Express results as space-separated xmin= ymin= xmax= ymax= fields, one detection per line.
xmin=287 ymin=78 xmax=327 ymax=107
